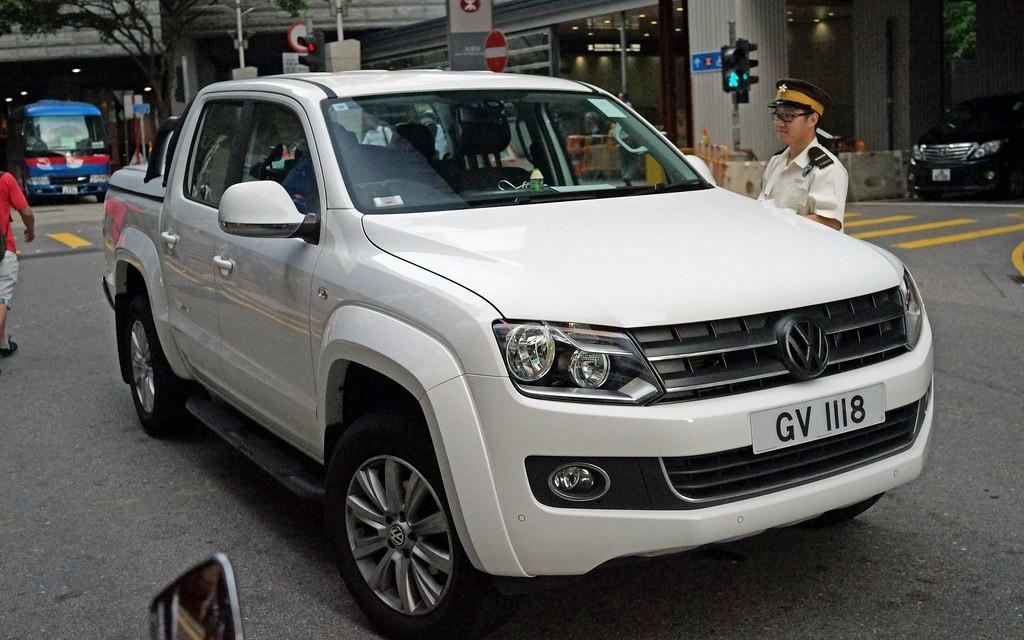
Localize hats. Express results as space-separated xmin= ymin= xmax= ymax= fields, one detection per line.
xmin=767 ymin=79 xmax=832 ymax=117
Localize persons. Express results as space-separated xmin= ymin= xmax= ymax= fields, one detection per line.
xmin=0 ymin=170 xmax=34 ymax=357
xmin=755 ymin=78 xmax=849 ymax=235
xmin=283 ymin=123 xmax=359 ymax=215
xmin=585 ymin=93 xmax=643 ymax=184
xmin=102 ymin=194 xmax=151 ymax=287
xmin=363 ymin=117 xmax=393 ymax=146
xmin=421 ymin=113 xmax=448 ymax=161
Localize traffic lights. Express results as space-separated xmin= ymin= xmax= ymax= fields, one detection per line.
xmin=738 ymin=41 xmax=760 ymax=93
xmin=720 ymin=45 xmax=745 ymax=93
xmin=298 ymin=31 xmax=324 ymax=72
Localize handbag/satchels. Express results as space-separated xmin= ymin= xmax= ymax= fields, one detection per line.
xmin=0 ymin=233 xmax=6 ymax=261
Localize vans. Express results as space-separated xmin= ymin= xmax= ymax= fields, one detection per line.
xmin=908 ymin=92 xmax=1024 ymax=201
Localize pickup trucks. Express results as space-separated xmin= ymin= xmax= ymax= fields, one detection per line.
xmin=100 ymin=67 xmax=937 ymax=640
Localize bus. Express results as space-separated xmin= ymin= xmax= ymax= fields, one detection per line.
xmin=7 ymin=98 xmax=110 ymax=203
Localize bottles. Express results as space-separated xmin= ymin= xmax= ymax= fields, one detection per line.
xmin=530 ymin=163 xmax=544 ymax=191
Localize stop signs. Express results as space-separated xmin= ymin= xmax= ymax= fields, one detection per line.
xmin=484 ymin=29 xmax=510 ymax=72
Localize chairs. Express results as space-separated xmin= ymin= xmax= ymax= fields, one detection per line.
xmin=451 ymin=109 xmax=531 ymax=194
xmin=568 ymin=91 xmax=624 ymax=175
xmin=390 ymin=124 xmax=447 ymax=187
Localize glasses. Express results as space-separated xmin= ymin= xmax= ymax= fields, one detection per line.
xmin=769 ymin=111 xmax=811 ymax=122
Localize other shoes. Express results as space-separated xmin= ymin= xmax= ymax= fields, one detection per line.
xmin=0 ymin=336 xmax=18 ymax=358
